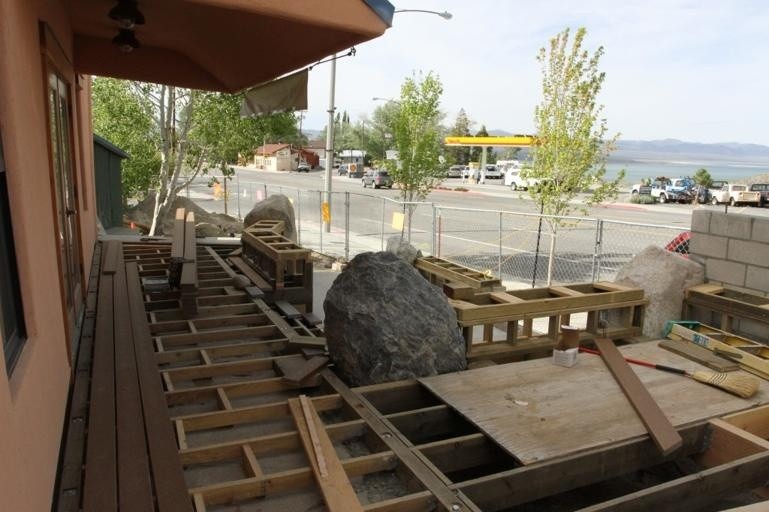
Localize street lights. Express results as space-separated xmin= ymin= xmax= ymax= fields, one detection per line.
xmin=370 ymin=97 xmax=401 ymax=107
xmin=324 ymin=8 xmax=453 ymax=231
xmin=296 ymin=109 xmax=308 ymax=162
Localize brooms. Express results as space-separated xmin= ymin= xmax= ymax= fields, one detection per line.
xmin=578 ymin=344 xmax=762 ymax=398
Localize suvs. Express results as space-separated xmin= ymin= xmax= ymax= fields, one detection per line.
xmin=297 ymin=163 xmax=310 ymax=174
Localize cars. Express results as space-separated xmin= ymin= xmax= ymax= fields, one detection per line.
xmin=338 ymin=163 xmax=348 ymax=176
xmin=443 ymin=159 xmax=549 ymax=192
xmin=632 ymin=177 xmax=769 ymax=210
xmin=361 ymin=170 xmax=393 ymax=190
xmin=666 ymin=232 xmax=691 ymax=260
